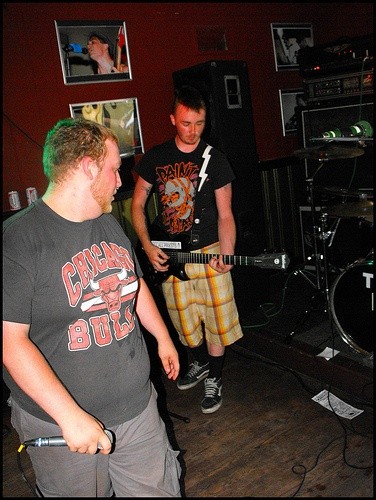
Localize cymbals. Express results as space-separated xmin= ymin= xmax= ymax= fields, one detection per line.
xmin=317 ymin=185 xmax=369 ymax=198
xmin=293 ymin=146 xmax=366 ymax=160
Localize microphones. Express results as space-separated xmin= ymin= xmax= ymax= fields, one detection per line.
xmin=63 ymin=44 xmax=88 ymax=55
xmin=24 ymin=430 xmax=113 ymax=450
xmin=318 ymin=121 xmax=374 ymax=141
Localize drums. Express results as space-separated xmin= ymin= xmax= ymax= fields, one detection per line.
xmin=321 ymin=201 xmax=376 ymax=268
xmin=330 ymin=261 xmax=376 ymax=359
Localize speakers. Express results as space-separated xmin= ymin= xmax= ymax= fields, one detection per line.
xmin=229 ymin=159 xmax=264 ymax=258
xmin=171 ymin=60 xmax=257 ymax=160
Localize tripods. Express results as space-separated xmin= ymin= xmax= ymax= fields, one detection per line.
xmin=284 ymin=157 xmax=329 ymax=346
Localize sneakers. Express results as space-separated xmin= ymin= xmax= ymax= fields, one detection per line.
xmin=176 ymin=360 xmax=211 ymax=390
xmin=200 ymin=377 xmax=224 ymax=414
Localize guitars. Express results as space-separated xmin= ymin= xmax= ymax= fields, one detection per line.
xmin=135 ymin=225 xmax=290 ymax=288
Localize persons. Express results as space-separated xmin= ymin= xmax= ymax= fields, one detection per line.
xmin=87 ymin=32 xmax=129 ymax=73
xmin=0 ymin=118 xmax=183 ymax=498
xmin=132 ymin=92 xmax=244 ymax=414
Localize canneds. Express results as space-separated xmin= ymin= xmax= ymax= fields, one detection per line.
xmin=7 ymin=190 xmax=22 ymax=211
xmin=26 ymin=187 xmax=38 ymax=206
